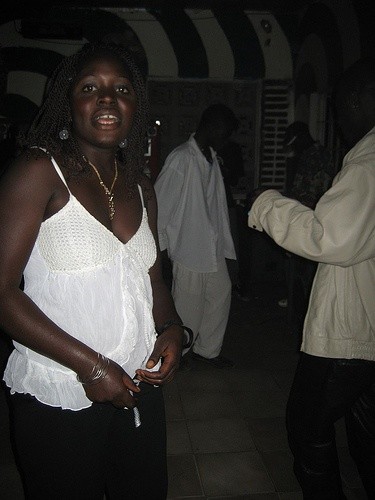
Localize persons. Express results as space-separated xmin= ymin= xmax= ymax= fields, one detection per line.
xmin=0 ymin=41 xmax=190 ymax=499
xmin=153 ymin=104 xmax=237 ymax=369
xmin=278 ymin=122 xmax=332 ymax=324
xmin=0 ymin=93 xmax=43 ymax=177
xmin=211 ymin=137 xmax=249 ymax=303
xmin=244 ymin=54 xmax=375 ymax=500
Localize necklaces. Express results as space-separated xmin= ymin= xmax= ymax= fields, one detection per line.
xmin=81 ymin=154 xmax=118 ymax=221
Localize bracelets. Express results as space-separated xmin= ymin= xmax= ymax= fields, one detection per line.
xmin=77 ymin=354 xmax=112 ymax=385
xmin=156 ymin=318 xmax=192 ymax=349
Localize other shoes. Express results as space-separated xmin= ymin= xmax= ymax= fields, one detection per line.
xmin=194 ymin=349 xmax=234 ymax=368
xmin=161 ymin=348 xmax=194 ymax=370
